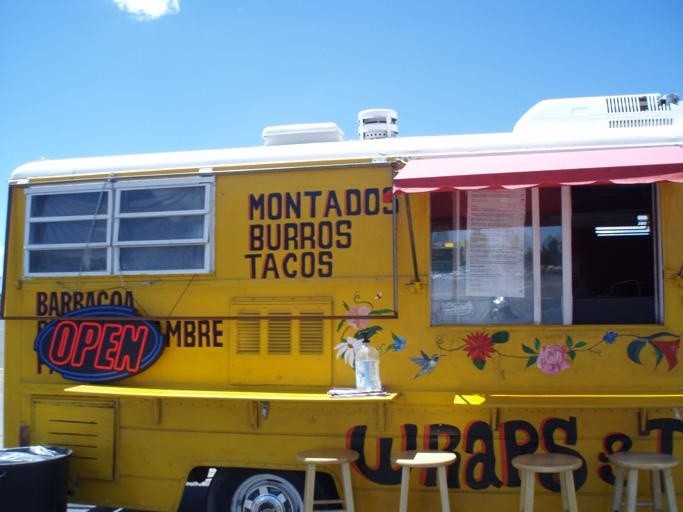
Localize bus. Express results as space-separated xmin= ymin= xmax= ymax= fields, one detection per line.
xmin=2 ymin=88 xmax=681 ymax=511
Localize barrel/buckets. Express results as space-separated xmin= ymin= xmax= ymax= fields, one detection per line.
xmin=0 ymin=445 xmax=73 ymax=511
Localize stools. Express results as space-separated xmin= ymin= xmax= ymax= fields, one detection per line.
xmin=298 ymin=450 xmax=359 ymax=512
xmin=609 ymin=453 xmax=678 ymax=510
xmin=512 ymin=453 xmax=583 ymax=511
xmin=392 ymin=451 xmax=457 ymax=510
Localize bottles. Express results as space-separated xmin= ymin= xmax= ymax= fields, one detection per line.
xmin=354 ymin=341 xmax=382 ymax=394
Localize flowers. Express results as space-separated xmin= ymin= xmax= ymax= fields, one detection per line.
xmin=395 ymin=337 xmax=406 ymax=350
xmin=463 ymin=332 xmax=495 ymax=363
xmin=536 ymin=345 xmax=570 ymax=375
xmin=652 ymin=339 xmax=680 ymax=370
xmin=334 ymin=337 xmax=370 ymax=365
xmin=344 ymin=306 xmax=370 ymax=329
xmin=603 ymin=332 xmax=617 ymax=343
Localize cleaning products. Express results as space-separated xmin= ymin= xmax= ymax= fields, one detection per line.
xmin=354 ymin=332 xmax=383 ymax=392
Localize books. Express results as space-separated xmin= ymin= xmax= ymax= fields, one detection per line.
xmin=327 ymin=387 xmax=391 ymax=398
